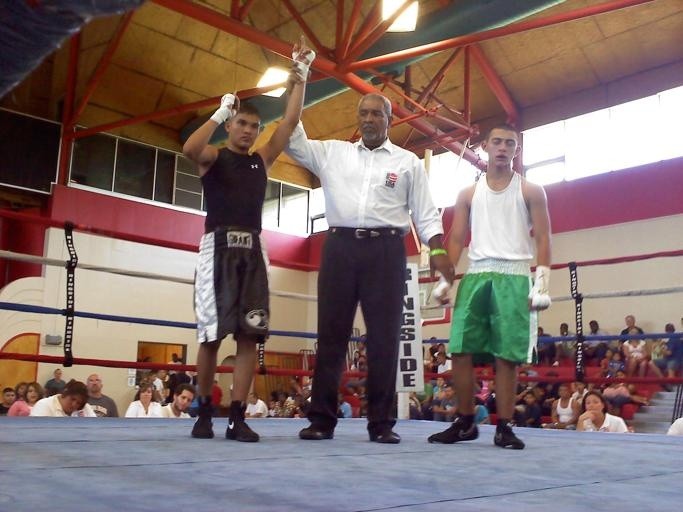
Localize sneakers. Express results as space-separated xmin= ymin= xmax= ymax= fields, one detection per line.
xmin=192 ymin=415 xmax=213 ymax=438
xmin=494 ymin=422 xmax=524 ymax=449
xmin=428 ymin=416 xmax=479 ymax=443
xmin=226 ymin=416 xmax=259 ymax=442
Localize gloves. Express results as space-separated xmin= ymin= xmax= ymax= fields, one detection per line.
xmin=431 ymin=270 xmax=452 ymax=306
xmin=292 ymin=34 xmax=316 ymax=82
xmin=210 ymin=93 xmax=240 ymax=125
xmin=527 ymin=265 xmax=551 ymax=311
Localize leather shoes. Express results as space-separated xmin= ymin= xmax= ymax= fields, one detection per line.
xmin=370 ymin=431 xmax=401 ymax=443
xmin=300 ymin=425 xmax=333 ymax=439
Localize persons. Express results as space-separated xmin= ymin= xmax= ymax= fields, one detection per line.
xmin=431 ymin=384 xmax=459 ymax=422
xmin=544 ymin=383 xmax=583 ymax=430
xmin=356 ymin=378 xmax=369 ymax=416
xmin=600 ymin=349 xmax=613 ymax=378
xmin=472 ymin=381 xmax=483 ymax=405
xmin=0 ymin=387 xmax=18 ymax=416
xmin=538 ymin=327 xmax=556 ymax=366
xmin=337 ymin=394 xmax=352 ymax=418
xmin=357 ymin=355 xmax=368 ymax=371
xmin=612 ymin=315 xmax=644 ymax=364
xmin=556 ymin=323 xmax=577 ymax=360
xmin=600 ymin=352 xmax=624 ymax=394
xmin=15 ymin=382 xmax=28 ymax=401
xmin=188 ymin=383 xmax=200 ymax=418
xmin=211 ymin=379 xmax=223 ymax=417
xmin=28 ymin=379 xmax=98 ymax=417
xmin=651 ymin=339 xmax=672 ymax=360
xmin=666 ymin=418 xmax=683 ymax=436
xmin=278 ymin=393 xmax=296 ymax=418
xmin=484 ymin=380 xmax=496 ymax=414
xmin=298 ymin=375 xmax=314 ymax=414
xmin=165 ymin=353 xmax=183 ymax=375
xmin=189 ymin=35 xmax=315 ymax=441
xmin=134 ymin=375 xmax=161 ymax=403
xmin=513 ymin=389 xmax=544 ymax=428
xmin=572 ymin=383 xmax=590 ymax=409
xmin=161 ymin=382 xmax=195 ymax=418
xmin=410 ymin=383 xmax=433 ymax=419
xmin=279 ymin=64 xmax=455 ymax=445
xmin=160 ymin=370 xmax=192 ymax=406
xmin=621 ymin=328 xmax=650 ymax=377
xmin=600 ymin=370 xmax=628 ymax=405
xmin=570 ymin=382 xmax=577 ymax=393
xmin=124 ymin=383 xmax=167 ymax=418
xmin=85 ymin=374 xmax=119 ymax=417
xmin=603 ymin=382 xmax=650 ymax=417
xmin=429 ymin=336 xmax=439 ymax=379
xmin=345 ymin=377 xmax=360 ymax=397
xmin=268 ymin=400 xmax=281 ymax=417
xmin=430 ymin=377 xmax=447 ymax=422
xmin=153 ymin=370 xmax=171 ymax=405
xmin=142 ymin=357 xmax=153 ymax=373
xmin=532 ymin=372 xmax=562 ymax=415
xmin=576 ymin=390 xmax=628 ymax=433
xmin=135 ymin=372 xmax=157 ymax=392
xmin=429 ymin=353 xmax=452 ymax=374
xmin=357 ymin=335 xmax=369 ymax=357
xmin=438 ymin=344 xmax=450 ymax=359
xmin=428 ymin=122 xmax=554 ymax=449
xmin=244 ymin=392 xmax=269 ymax=417
xmin=44 ymin=369 xmax=66 ymax=398
xmin=647 ymin=323 xmax=683 ymax=379
xmin=7 ymin=382 xmax=45 ymax=416
xmin=516 ymin=371 xmax=532 ymax=405
xmin=352 ymin=351 xmax=361 ymax=369
xmin=471 ymin=398 xmax=491 ymax=425
xmin=581 ymin=320 xmax=611 ymax=360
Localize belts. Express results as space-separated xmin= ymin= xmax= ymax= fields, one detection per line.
xmin=328 ymin=227 xmax=403 ymax=240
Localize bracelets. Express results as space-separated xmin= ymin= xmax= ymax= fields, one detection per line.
xmin=428 ymin=249 xmax=448 ymax=256
